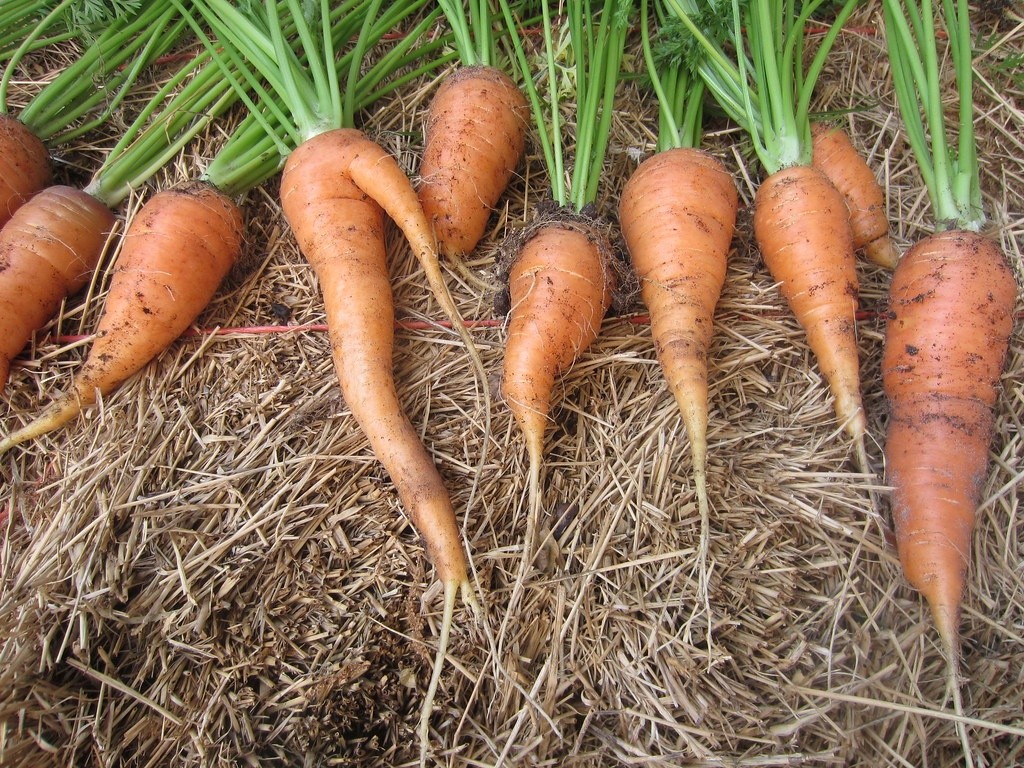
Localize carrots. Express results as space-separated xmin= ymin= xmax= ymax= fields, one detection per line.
xmin=0 ymin=64 xmax=1015 ymax=768
